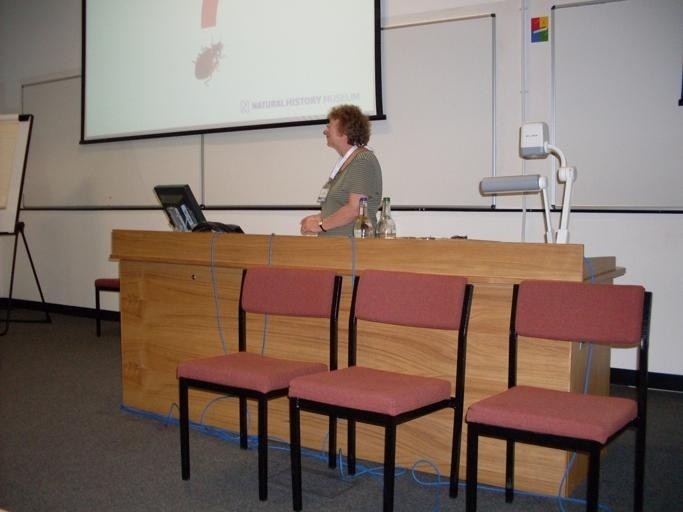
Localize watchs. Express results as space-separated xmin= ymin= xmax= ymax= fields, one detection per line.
xmin=318 ymin=220 xmax=327 ymax=232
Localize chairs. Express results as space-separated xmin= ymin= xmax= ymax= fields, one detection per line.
xmin=176 ymin=266 xmax=343 ymax=501
xmin=95 ymin=278 xmax=120 ymax=336
xmin=465 ymin=280 xmax=653 ymax=512
xmin=287 ymin=269 xmax=474 ymax=511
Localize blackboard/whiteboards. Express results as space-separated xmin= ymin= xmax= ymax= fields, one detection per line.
xmin=0 ymin=114 xmax=34 ymax=235
xmin=79 ymin=0 xmax=387 ymax=146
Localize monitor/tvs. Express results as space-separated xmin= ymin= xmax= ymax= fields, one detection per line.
xmin=154 ymin=184 xmax=207 ymax=232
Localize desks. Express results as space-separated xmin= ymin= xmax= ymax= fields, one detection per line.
xmin=110 ymin=229 xmax=627 ymax=498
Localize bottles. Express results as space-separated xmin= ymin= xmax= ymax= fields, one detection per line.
xmin=354 ymin=197 xmax=373 ymax=239
xmin=376 ymin=196 xmax=397 ymax=240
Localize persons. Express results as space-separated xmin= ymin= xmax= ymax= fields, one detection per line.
xmin=300 ymin=105 xmax=382 ymax=235
